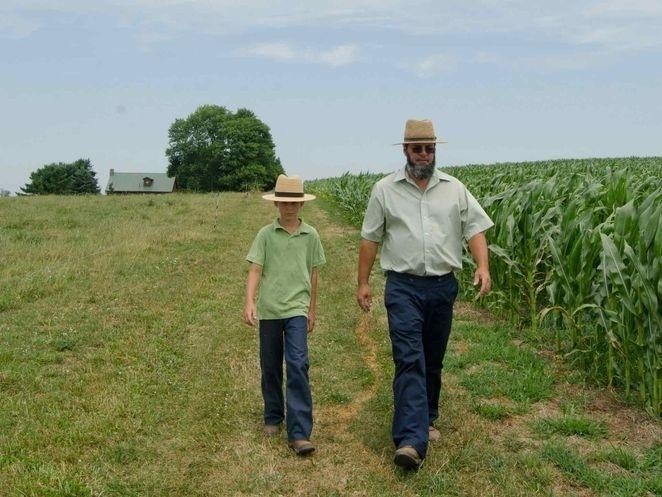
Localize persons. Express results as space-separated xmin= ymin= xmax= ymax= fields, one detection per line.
xmin=243 ymin=175 xmax=326 ymax=456
xmin=356 ymin=118 xmax=495 ymax=468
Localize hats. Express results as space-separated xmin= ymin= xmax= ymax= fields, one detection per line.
xmin=391 ymin=118 xmax=448 ymax=146
xmin=261 ymin=173 xmax=317 ymax=203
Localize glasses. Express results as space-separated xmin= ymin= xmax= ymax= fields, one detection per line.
xmin=408 ymin=145 xmax=436 ymax=156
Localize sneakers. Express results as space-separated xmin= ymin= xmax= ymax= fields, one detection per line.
xmin=428 ymin=425 xmax=441 ymax=441
xmin=262 ymin=424 xmax=281 ymax=438
xmin=287 ymin=439 xmax=316 ymax=455
xmin=393 ymin=444 xmax=423 ymax=474
